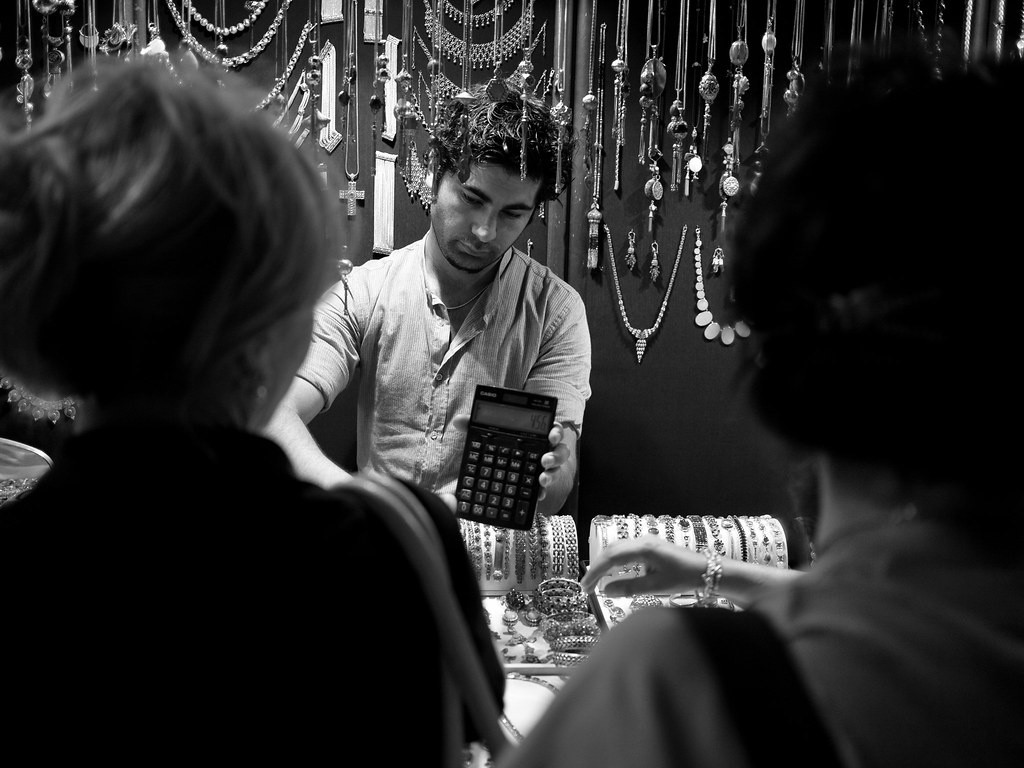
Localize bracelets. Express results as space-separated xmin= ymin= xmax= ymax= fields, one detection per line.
xmin=452 ymin=509 xmax=790 ymax=744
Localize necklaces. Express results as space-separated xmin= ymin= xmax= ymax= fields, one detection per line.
xmin=1 ymin=1 xmax=1024 ymax=363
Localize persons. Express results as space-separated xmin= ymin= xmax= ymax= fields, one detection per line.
xmin=581 ymin=539 xmax=804 ymax=611
xmin=257 ymin=96 xmax=592 ymax=537
xmin=482 ymin=42 xmax=1024 ymax=768
xmin=2 ymin=57 xmax=503 ymax=768
